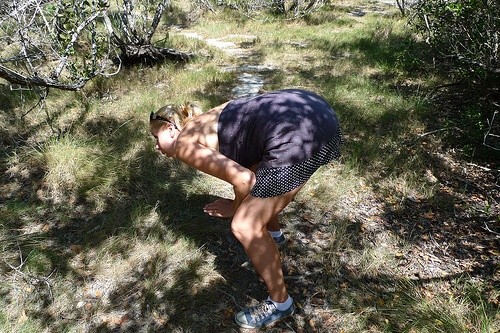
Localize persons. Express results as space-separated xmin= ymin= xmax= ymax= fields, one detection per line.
xmin=150 ymin=89 xmax=343 ymax=329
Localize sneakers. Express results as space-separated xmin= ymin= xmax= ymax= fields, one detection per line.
xmin=234 ymin=300 xmax=295 ymax=328
xmin=273 ymin=232 xmax=287 ymax=244
